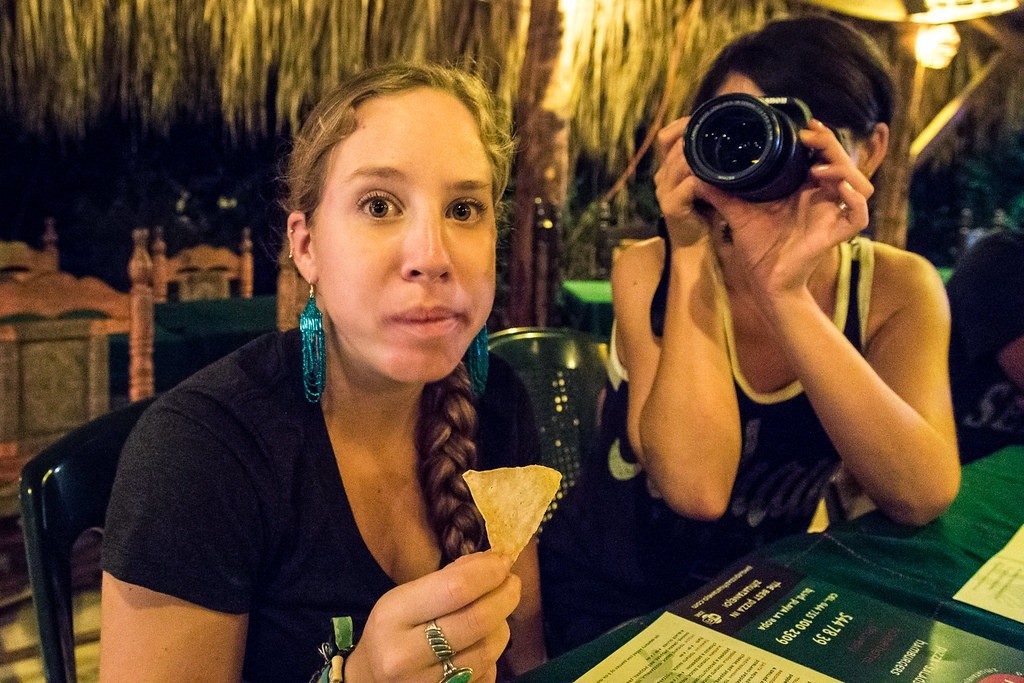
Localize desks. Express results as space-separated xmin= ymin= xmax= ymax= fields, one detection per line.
xmin=0 ymin=294 xmax=279 ymax=591
xmin=510 ymin=443 xmax=1024 ymax=683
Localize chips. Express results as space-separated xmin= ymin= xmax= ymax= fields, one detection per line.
xmin=461 ymin=465 xmax=562 ymax=568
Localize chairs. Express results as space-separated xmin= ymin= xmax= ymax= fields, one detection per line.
xmin=18 ymin=397 xmax=159 ymax=683
xmin=154 ymin=228 xmax=253 ymax=303
xmin=0 ymin=229 xmax=153 ymax=520
xmin=0 ymin=219 xmax=59 ymax=321
xmin=473 ymin=326 xmax=620 ymax=630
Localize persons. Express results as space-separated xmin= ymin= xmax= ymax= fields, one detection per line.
xmin=101 ymin=63 xmax=549 ymax=683
xmin=536 ymin=14 xmax=960 ymax=661
xmin=945 ymin=222 xmax=1024 ymax=465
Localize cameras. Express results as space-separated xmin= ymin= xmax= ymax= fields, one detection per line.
xmin=682 ymin=91 xmax=823 ymax=203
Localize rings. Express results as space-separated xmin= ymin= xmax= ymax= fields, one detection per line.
xmin=836 ymin=198 xmax=848 ymax=211
xmin=424 ymin=620 xmax=456 ymax=661
xmin=439 ymin=659 xmax=473 ymax=683
